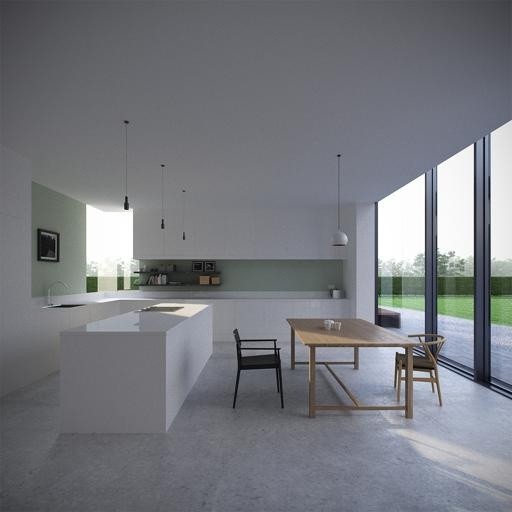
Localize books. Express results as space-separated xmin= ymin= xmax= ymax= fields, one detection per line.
xmin=157 ymin=264 xmax=176 ymax=272
xmin=148 ymin=274 xmax=167 ymax=285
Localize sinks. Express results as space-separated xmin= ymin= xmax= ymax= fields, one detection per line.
xmin=42 ymin=304 xmax=86 ymax=308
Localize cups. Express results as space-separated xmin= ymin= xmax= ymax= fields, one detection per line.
xmin=324 ymin=317 xmax=341 ymax=332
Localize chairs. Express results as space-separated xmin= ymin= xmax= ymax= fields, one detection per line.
xmin=232 ymin=328 xmax=284 ymax=409
xmin=394 ymin=333 xmax=448 ymax=407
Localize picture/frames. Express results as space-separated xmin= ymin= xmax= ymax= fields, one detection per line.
xmin=37 ymin=228 xmax=60 ymax=262
xmin=204 ymin=261 xmax=216 ymax=272
xmin=192 ymin=261 xmax=204 ymax=272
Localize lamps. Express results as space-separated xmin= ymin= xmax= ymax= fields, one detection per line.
xmin=123 ymin=119 xmax=130 ymax=210
xmin=182 ymin=189 xmax=186 ymax=240
xmin=160 ymin=164 xmax=165 ymax=229
xmin=330 ymin=154 xmax=348 ymax=246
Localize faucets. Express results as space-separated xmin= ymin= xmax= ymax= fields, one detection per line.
xmin=47 ymin=280 xmax=68 ymax=306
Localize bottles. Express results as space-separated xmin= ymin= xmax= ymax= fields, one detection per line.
xmin=149 ymin=272 xmax=167 ymax=285
